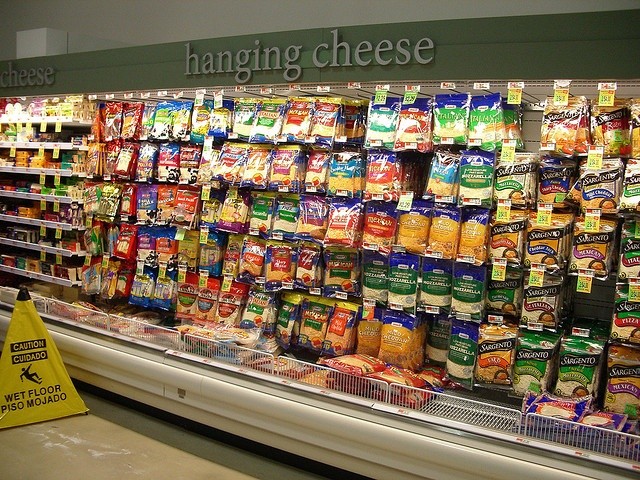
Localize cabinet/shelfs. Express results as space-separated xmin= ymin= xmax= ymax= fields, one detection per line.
xmin=4 ymin=98 xmax=640 ymax=480
